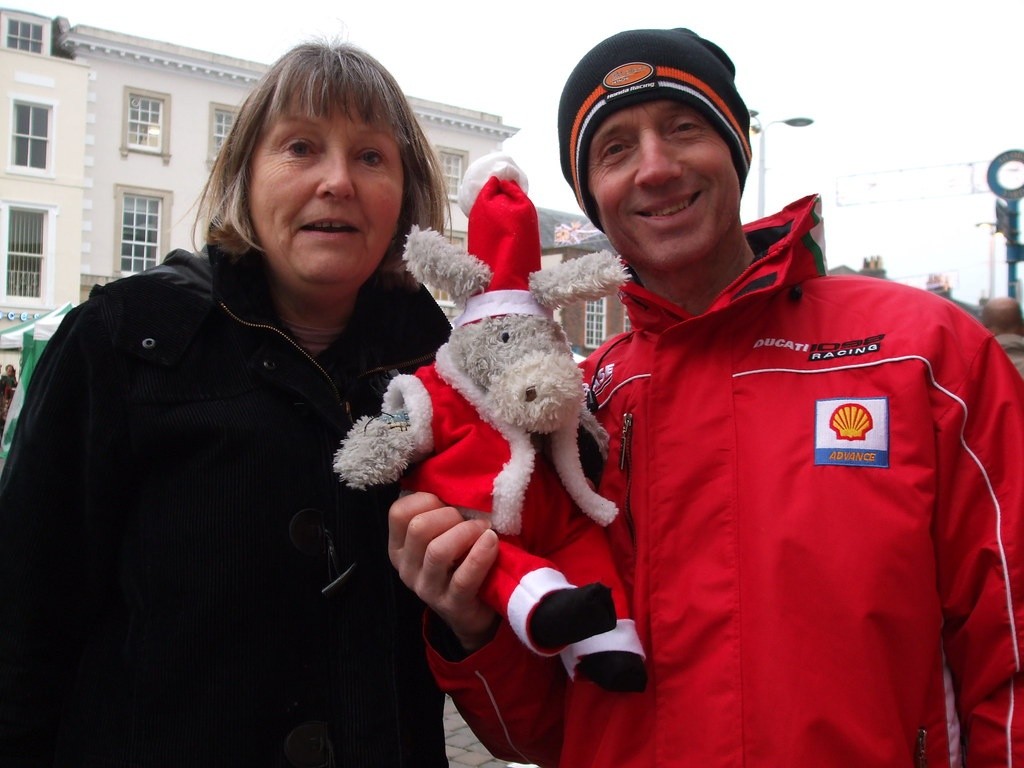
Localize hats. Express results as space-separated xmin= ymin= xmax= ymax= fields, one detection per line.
xmin=558 ymin=28 xmax=753 ymax=233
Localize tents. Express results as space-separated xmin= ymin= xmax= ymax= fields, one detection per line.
xmin=0 ymin=301 xmax=72 ymax=461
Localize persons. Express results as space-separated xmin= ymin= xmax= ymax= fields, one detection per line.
xmin=976 ymin=294 xmax=1024 ymax=383
xmin=0 ymin=365 xmax=18 ymax=435
xmin=386 ymin=27 xmax=1024 ymax=768
xmin=0 ymin=39 xmax=463 ymax=768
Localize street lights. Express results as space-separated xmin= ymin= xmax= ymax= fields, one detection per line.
xmin=974 ymin=222 xmax=1001 ymax=298
xmin=748 ymin=107 xmax=816 ymax=220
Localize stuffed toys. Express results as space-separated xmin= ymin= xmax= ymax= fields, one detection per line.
xmin=332 ymin=152 xmax=651 ymax=695
